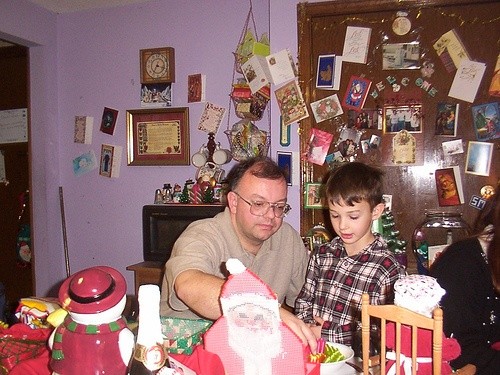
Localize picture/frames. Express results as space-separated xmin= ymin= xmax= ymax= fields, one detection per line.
xmin=382 ymin=103 xmax=423 ymax=135
xmin=125 ymin=107 xmax=190 ymax=166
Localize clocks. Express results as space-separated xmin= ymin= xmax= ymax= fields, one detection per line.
xmin=139 ymin=46 xmax=175 ymax=84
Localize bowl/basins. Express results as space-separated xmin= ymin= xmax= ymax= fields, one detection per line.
xmin=318 ymin=341 xmax=354 ymax=375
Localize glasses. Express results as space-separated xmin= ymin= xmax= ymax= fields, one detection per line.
xmin=233 ymin=191 xmax=291 ymax=218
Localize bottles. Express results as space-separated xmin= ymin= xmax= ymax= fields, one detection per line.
xmin=411 ymin=212 xmax=472 ymax=271
xmin=125 ymin=284 xmax=174 ymax=375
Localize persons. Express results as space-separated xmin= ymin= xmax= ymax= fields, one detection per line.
xmin=427 ymin=188 xmax=500 ymax=375
xmin=295 ymin=162 xmax=403 ymax=357
xmin=341 ymin=107 xmax=456 ymax=156
xmin=385 ymin=274 xmax=460 ymax=375
xmin=162 ymin=157 xmax=320 ymax=357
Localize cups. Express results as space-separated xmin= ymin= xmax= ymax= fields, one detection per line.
xmin=191 ymin=145 xmax=210 ymax=167
xmin=212 ymin=144 xmax=233 ymax=165
xmin=197 ymin=162 xmax=225 ymax=184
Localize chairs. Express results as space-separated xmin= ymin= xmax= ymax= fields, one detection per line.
xmin=360 ymin=293 xmax=444 ymax=375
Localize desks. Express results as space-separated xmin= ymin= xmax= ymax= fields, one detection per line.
xmin=127 ymin=261 xmax=165 ymax=300
